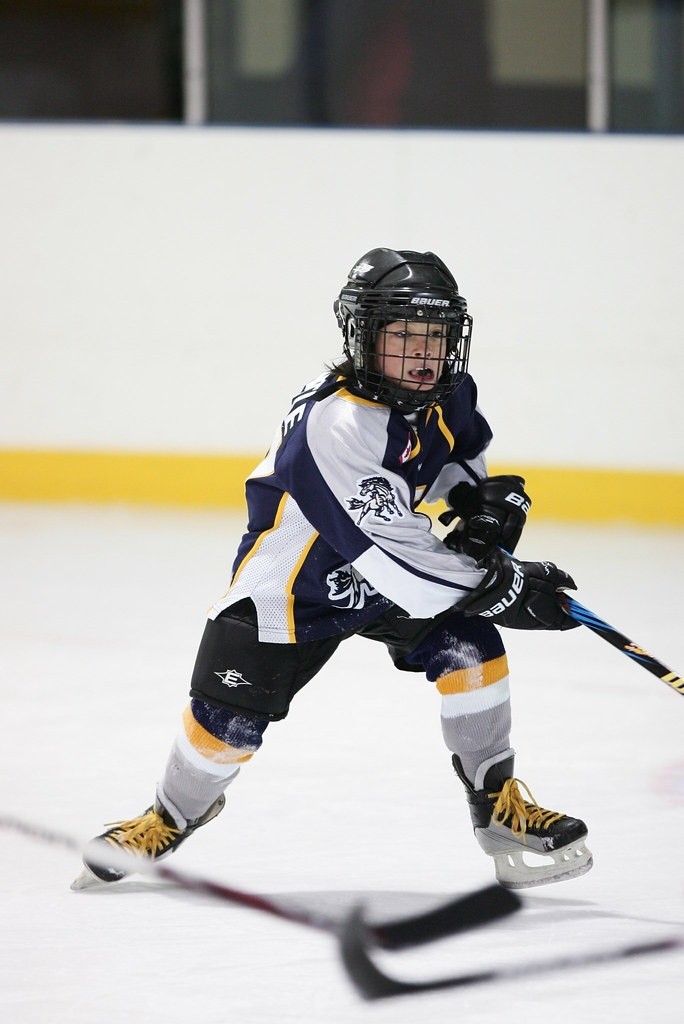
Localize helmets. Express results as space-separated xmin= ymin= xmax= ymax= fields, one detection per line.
xmin=334 ymin=248 xmax=473 ymax=414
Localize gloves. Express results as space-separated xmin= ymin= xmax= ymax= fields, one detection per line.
xmin=455 ymin=545 xmax=582 ymax=631
xmin=439 ymin=475 xmax=533 ymax=561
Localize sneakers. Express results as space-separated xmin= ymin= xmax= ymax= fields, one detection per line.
xmin=72 ymin=781 xmax=225 ymax=889
xmin=451 ymin=748 xmax=594 ymax=889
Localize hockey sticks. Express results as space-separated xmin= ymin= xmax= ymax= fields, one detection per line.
xmin=495 ymin=541 xmax=683 ymax=702
xmin=330 ymin=893 xmax=684 ymax=1005
xmin=0 ymin=811 xmax=531 ymax=958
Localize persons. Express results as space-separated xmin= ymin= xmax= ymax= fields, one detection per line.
xmin=82 ymin=248 xmax=588 ymax=884
